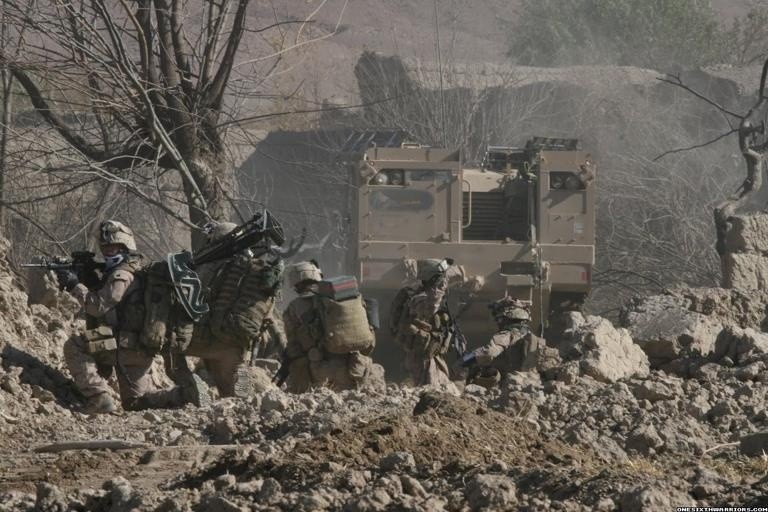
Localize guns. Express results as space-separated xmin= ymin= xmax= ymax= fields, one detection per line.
xmin=271 ymin=347 xmax=292 ymax=386
xmin=20 ymin=250 xmax=108 ymax=290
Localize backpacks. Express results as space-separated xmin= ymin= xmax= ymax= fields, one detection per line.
xmin=315 ymin=293 xmax=373 ymax=355
xmin=142 ymin=262 xmax=196 ymax=353
xmin=206 ymin=247 xmax=286 ymax=349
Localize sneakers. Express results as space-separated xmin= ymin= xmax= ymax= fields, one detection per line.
xmin=185 ymin=372 xmax=214 ymax=410
xmin=74 ymin=391 xmax=122 ymax=416
xmin=228 ymin=363 xmax=252 ymax=400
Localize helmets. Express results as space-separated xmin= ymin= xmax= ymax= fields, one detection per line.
xmin=97 ymin=219 xmax=137 ymax=254
xmin=417 ymin=256 xmax=449 ymax=283
xmin=196 ymin=222 xmax=242 ymax=259
xmin=290 ymin=260 xmax=323 ymax=291
xmin=489 ymin=295 xmax=531 ymax=323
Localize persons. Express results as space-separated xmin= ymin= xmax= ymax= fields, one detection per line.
xmin=474 ymin=295 xmax=544 ymax=396
xmin=199 ymin=220 xmax=275 ymax=398
xmin=54 ymin=221 xmax=210 ymax=414
xmin=391 ymin=257 xmax=466 ymax=385
xmin=283 ymin=261 xmax=372 ymax=392
